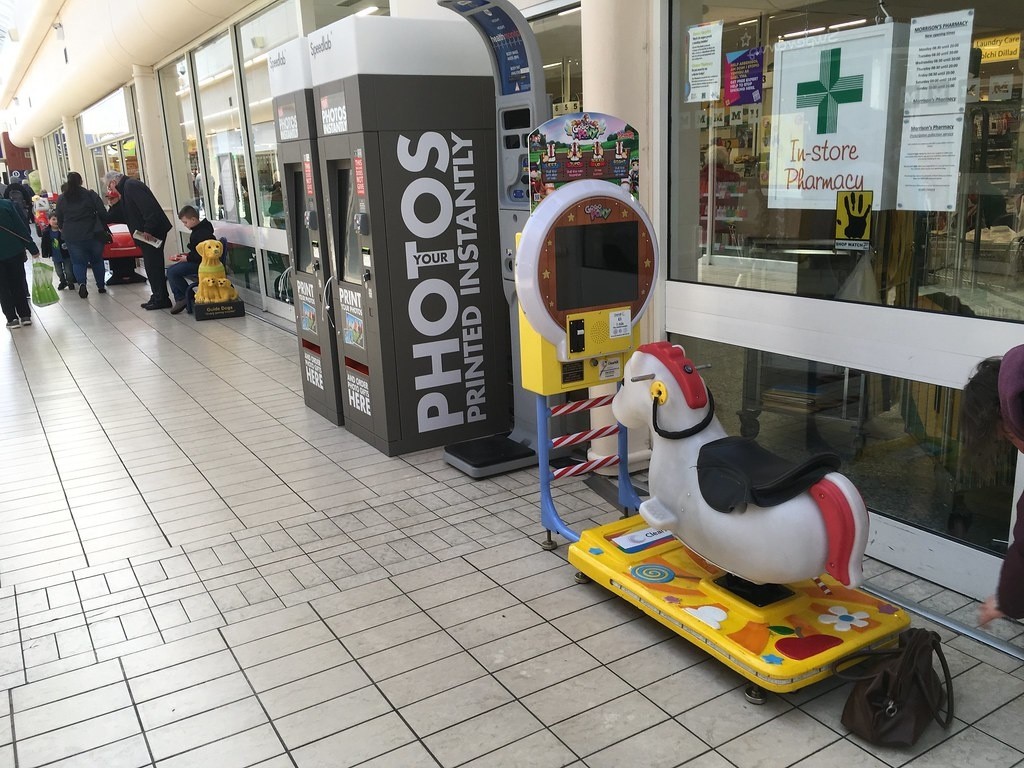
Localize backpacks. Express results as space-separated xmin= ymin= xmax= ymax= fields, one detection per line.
xmin=7 ymin=184 xmax=25 ymax=211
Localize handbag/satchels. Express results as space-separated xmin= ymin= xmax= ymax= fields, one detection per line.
xmin=90 ymin=189 xmax=113 ymax=244
xmin=834 ymin=627 xmax=954 ymax=750
xmin=32 ymin=255 xmax=59 ymax=307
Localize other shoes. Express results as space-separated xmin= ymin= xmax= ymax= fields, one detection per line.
xmin=170 ymin=294 xmax=195 ymax=314
xmin=68 ymin=282 xmax=75 ymax=290
xmin=79 ymin=284 xmax=88 ymax=298
xmin=58 ymin=282 xmax=67 ymax=290
xmin=146 ymin=297 xmax=173 ymax=311
xmin=98 ymin=287 xmax=106 ymax=293
xmin=141 ymin=294 xmax=155 ymax=308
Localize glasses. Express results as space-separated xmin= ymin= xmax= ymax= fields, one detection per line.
xmin=107 ymin=180 xmax=113 ymax=191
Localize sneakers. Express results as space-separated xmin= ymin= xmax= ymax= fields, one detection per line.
xmin=6 ymin=319 xmax=20 ymax=329
xmin=21 ymin=316 xmax=31 ymax=325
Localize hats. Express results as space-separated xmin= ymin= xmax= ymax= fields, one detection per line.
xmin=105 ymin=191 xmax=119 ymax=198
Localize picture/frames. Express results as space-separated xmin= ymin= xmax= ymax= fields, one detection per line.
xmin=216 ymin=152 xmax=240 ymax=223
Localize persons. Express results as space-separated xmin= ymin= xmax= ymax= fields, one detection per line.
xmin=700 ymin=145 xmax=740 ymax=245
xmin=105 ymin=170 xmax=173 ymax=310
xmin=0 ymin=199 xmax=40 ymax=328
xmin=269 ymin=181 xmax=287 ymax=228
xmin=0 ymin=171 xmax=109 ymax=299
xmin=167 ymin=206 xmax=217 ymax=314
xmin=961 ymin=344 xmax=1024 ymax=630
xmin=191 ymin=172 xmax=215 ymax=216
